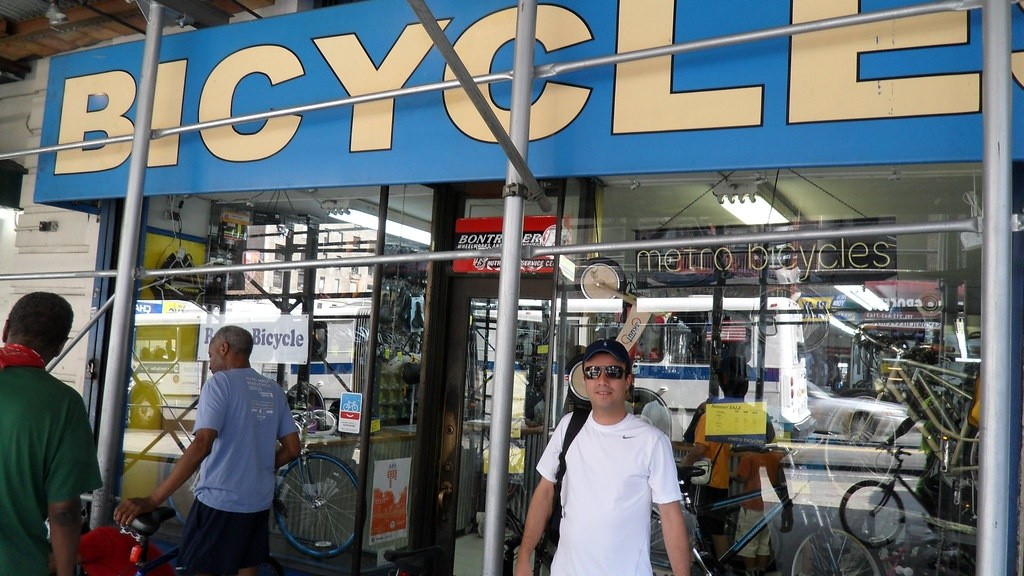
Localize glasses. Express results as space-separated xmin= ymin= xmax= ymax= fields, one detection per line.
xmin=583 ymin=365 xmax=626 ymax=380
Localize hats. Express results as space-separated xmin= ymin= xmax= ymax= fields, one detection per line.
xmin=582 ymin=340 xmax=631 ymax=375
xmin=715 ymin=356 xmax=747 ymax=377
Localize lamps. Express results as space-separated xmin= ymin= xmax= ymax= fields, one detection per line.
xmin=712 ymin=178 xmax=798 ymax=227
xmin=46 ymin=0 xmax=68 ymax=26
xmin=326 ymin=199 xmax=430 ymax=246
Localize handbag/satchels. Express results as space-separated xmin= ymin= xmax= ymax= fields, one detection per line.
xmin=544 ymin=484 xmax=563 ymax=554
xmin=690 ymin=458 xmax=712 ymax=484
xmin=76 ymin=526 xmax=174 ymax=576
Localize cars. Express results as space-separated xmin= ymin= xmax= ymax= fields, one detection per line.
xmin=807 ymin=379 xmax=922 ymax=447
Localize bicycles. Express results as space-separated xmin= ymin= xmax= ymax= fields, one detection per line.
xmin=650 ymin=448 xmax=888 ymax=576
xmin=118 ymin=506 xmax=287 ymax=576
xmin=161 ymin=402 xmax=369 ymax=558
xmin=838 ymin=443 xmax=978 ymax=549
xmin=824 ymin=325 xmax=978 ymax=500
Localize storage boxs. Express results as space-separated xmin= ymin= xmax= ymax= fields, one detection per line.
xmin=455 ymin=217 xmax=572 ymax=271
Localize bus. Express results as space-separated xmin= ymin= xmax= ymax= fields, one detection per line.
xmin=123 ymin=296 xmax=816 ymax=471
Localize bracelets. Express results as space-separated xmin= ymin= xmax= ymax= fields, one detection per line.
xmin=783 ymin=499 xmax=794 ymax=508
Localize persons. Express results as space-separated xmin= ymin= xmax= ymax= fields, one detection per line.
xmin=624 ymin=384 xmax=635 ymax=416
xmin=514 ymin=339 xmax=690 ymax=576
xmin=642 ymin=373 xmax=688 ymax=442
xmin=729 ymin=422 xmax=784 ymax=576
xmin=533 ymin=376 xmax=573 ymax=429
xmin=0 ymin=292 xmax=102 ymax=576
xmin=113 ymin=328 xmax=301 ymax=576
xmin=684 ymin=356 xmax=794 ymax=570
xmin=476 ymin=411 xmax=544 ymax=539
xmin=877 ymin=376 xmax=960 ymax=556
